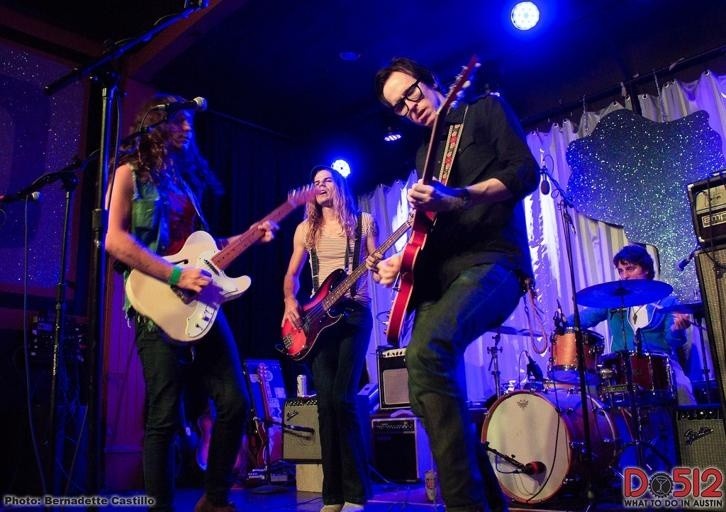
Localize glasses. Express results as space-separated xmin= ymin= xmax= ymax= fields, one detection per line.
xmin=394 ymin=80 xmax=422 ymax=117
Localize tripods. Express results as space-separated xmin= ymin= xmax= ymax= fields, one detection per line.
xmin=531 ymin=176 xmax=634 ymax=512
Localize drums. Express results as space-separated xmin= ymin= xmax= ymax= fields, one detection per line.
xmin=598 ymin=350 xmax=676 ymax=407
xmin=481 ymin=381 xmax=618 ymax=505
xmin=552 ymin=327 xmax=599 ymax=372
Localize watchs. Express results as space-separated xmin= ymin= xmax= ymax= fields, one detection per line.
xmin=460 ymin=187 xmax=472 ymax=212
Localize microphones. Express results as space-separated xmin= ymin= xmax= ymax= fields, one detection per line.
xmin=525 ymin=461 xmax=546 ymax=475
xmin=289 ymin=426 xmax=314 ymax=432
xmin=541 ymin=173 xmax=550 ymax=194
xmin=677 ymin=252 xmax=695 ymax=271
xmin=153 ymin=96 xmax=208 ymax=111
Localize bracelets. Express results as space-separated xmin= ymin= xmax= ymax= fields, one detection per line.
xmin=169 ymin=266 xmax=182 ymax=286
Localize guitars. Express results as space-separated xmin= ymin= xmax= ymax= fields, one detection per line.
xmin=384 ymin=56 xmax=476 ymax=348
xmin=194 ymin=414 xmax=242 ymax=474
xmin=241 ymin=358 xmax=283 ymax=468
xmin=125 ymin=184 xmax=321 ymax=342
xmin=282 ymin=209 xmax=416 ymax=361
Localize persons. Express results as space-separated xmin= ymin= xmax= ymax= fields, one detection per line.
xmin=280 ymin=167 xmax=385 ymax=511
xmin=373 ymin=57 xmax=540 ymax=511
xmin=105 ymin=95 xmax=279 ymax=511
xmin=566 ymin=245 xmax=698 ymax=405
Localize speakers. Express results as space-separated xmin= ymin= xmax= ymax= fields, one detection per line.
xmin=371 ymin=418 xmax=433 ymax=483
xmin=283 ymin=399 xmax=324 ymax=460
xmin=378 ymin=346 xmax=414 ymax=410
xmin=673 ymin=404 xmax=726 ymax=512
xmin=695 ymin=244 xmax=726 ymax=413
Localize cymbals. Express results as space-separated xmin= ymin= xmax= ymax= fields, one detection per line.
xmin=488 ymin=326 xmax=542 ymax=336
xmin=658 ymin=300 xmax=702 ymax=313
xmin=574 ymin=279 xmax=673 ymax=309
